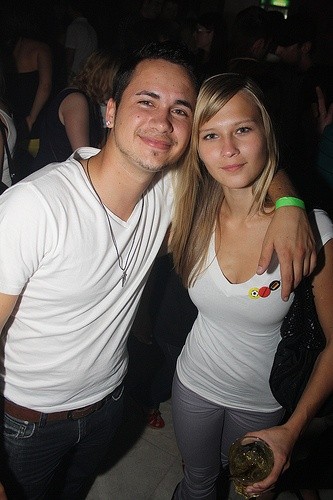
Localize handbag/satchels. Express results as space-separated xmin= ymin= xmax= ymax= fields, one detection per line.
xmin=1 ymin=120 xmax=18 ymax=193
xmin=267 ymin=280 xmax=332 ymax=418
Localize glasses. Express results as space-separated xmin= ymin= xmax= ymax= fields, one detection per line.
xmin=193 ymin=27 xmax=212 ymax=34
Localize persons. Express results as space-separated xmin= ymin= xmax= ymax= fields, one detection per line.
xmin=0 ymin=0 xmax=333 ymax=500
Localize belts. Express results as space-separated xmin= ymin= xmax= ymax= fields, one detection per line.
xmin=1 ymin=393 xmax=113 ymax=425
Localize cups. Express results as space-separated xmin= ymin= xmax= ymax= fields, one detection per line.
xmin=228 ymin=436 xmax=274 ymax=498
